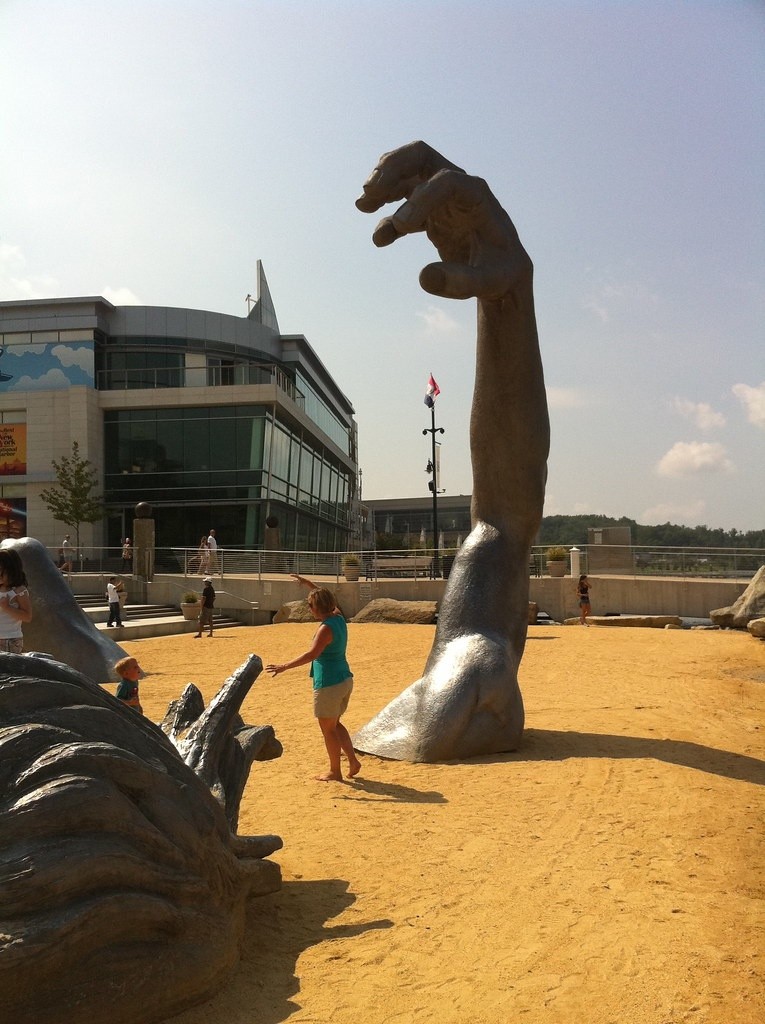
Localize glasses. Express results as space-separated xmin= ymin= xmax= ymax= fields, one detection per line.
xmin=308 ymin=602 xmax=316 ymax=608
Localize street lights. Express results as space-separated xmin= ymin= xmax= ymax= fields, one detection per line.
xmin=423 ymin=406 xmax=445 ymax=577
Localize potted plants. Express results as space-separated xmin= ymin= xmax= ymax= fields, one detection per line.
xmin=546 ymin=548 xmax=568 ymax=578
xmin=340 ymin=554 xmax=360 ymax=582
xmin=181 ymin=590 xmax=203 ymax=621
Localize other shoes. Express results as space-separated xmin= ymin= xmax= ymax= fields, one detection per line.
xmin=107 ymin=624 xmax=114 ymax=627
xmin=116 ymin=623 xmax=124 ymax=627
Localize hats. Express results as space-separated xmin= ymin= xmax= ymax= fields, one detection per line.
xmin=203 ymin=576 xmax=213 ymax=582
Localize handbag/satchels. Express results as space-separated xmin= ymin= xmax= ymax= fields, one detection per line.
xmin=198 ymin=548 xmax=205 ymax=555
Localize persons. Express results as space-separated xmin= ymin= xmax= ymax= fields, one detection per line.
xmin=120 ymin=538 xmax=133 ymax=574
xmin=115 ymin=657 xmax=143 ymax=713
xmin=0 ymin=549 xmax=32 ymax=653
xmin=0 ymin=140 xmax=549 ymax=1024
xmin=264 ymin=575 xmax=361 ymax=781
xmin=193 ymin=577 xmax=216 ymax=638
xmin=106 ymin=577 xmax=124 ymax=627
xmin=58 ymin=535 xmax=75 ymax=575
xmin=196 ymin=530 xmax=224 ymax=576
xmin=576 ymin=575 xmax=593 ymax=627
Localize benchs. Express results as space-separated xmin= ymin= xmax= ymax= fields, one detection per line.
xmin=366 ymin=555 xmax=436 ymax=581
xmin=530 ymin=554 xmax=540 ymax=577
